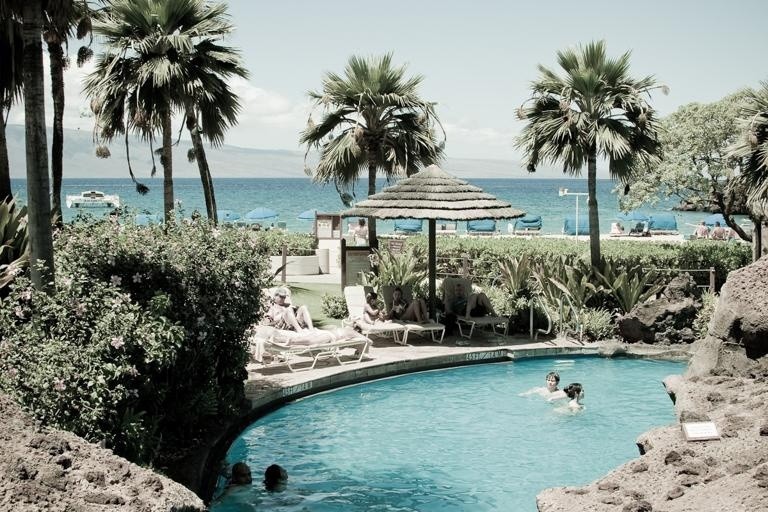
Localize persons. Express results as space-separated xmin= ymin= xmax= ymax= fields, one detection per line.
xmin=694 ymin=219 xmax=708 ymax=238
xmin=210 ymin=462 xmax=252 ymax=508
xmin=386 ymin=287 xmax=431 ymax=324
xmin=449 ymin=283 xmax=496 ymax=320
xmin=518 ymin=371 xmax=567 ymax=403
xmin=261 ymin=464 xmax=306 ymax=508
xmin=555 ymin=383 xmax=586 ymax=416
xmin=362 ymin=292 xmax=387 ymax=325
xmin=352 ymin=217 xmax=368 ymax=246
xmin=264 ymin=325 xmax=372 ymax=347
xmin=709 ymin=221 xmax=725 ymax=241
xmin=264 ymin=288 xmax=314 ymax=334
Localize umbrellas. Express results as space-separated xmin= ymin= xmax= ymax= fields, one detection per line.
xmin=243 ymin=206 xmax=280 ymax=231
xmin=339 ymin=163 xmax=525 ymax=322
xmin=202 ymin=208 xmax=242 ymax=222
xmin=296 ymin=208 xmax=327 ymax=232
xmin=616 ymin=209 xmax=647 ymax=233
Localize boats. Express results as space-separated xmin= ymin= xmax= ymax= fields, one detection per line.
xmin=65 ymin=190 xmax=119 ymax=208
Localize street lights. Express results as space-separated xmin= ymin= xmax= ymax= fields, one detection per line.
xmin=558 ymin=188 xmax=589 ymax=246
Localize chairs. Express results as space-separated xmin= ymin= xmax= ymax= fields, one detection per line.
xmin=244 ymin=279 xmax=511 ymax=373
